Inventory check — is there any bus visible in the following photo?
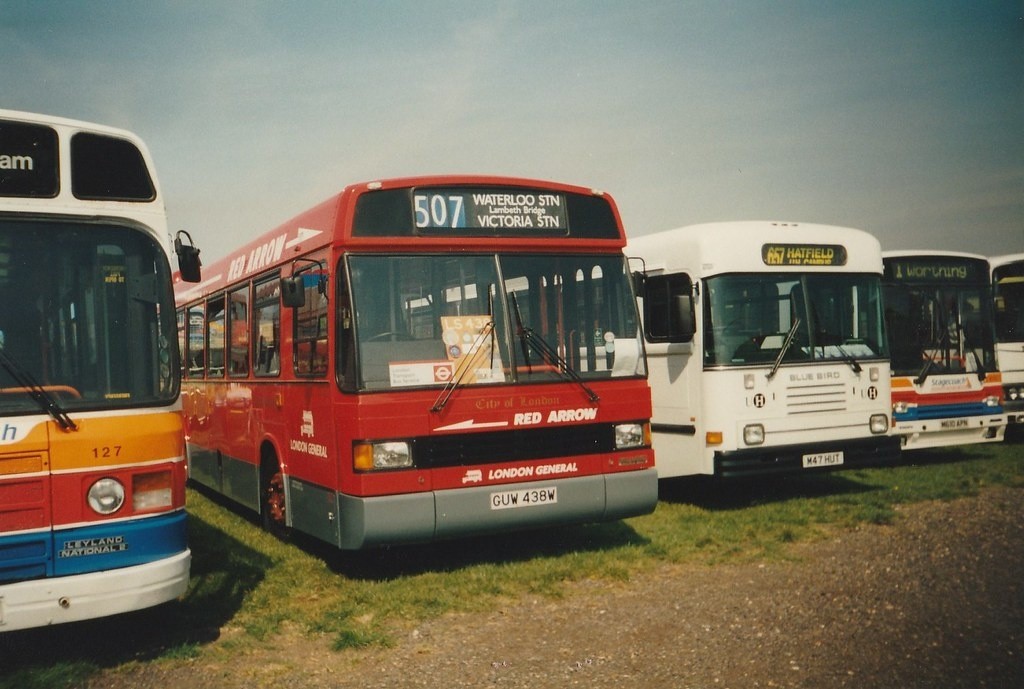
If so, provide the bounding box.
[155,175,659,552]
[402,218,893,483]
[780,249,1024,456]
[0,110,203,632]
